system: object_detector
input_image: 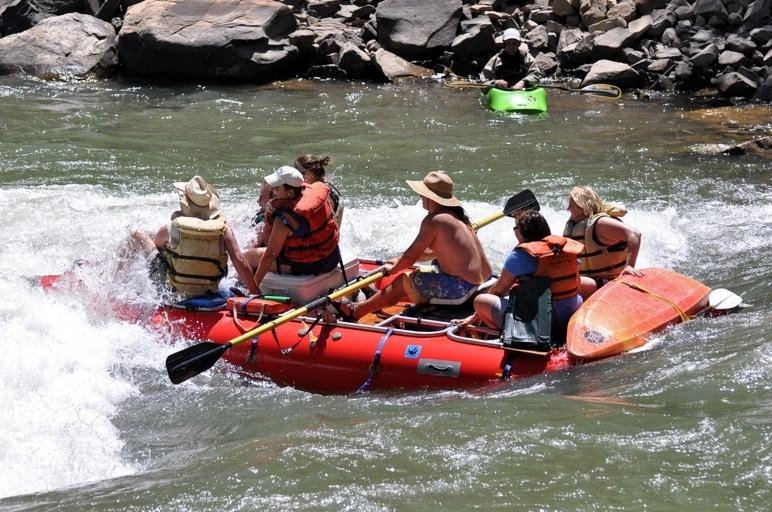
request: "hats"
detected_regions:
[263,165,305,188]
[405,168,463,210]
[172,174,220,219]
[502,26,523,43]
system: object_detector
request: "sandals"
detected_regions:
[356,276,377,299]
[328,287,360,324]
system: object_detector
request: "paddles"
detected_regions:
[442,79,621,99]
[689,287,743,318]
[164,188,542,383]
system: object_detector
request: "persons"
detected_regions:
[243,154,344,293]
[127,176,261,308]
[480,28,541,92]
[472,209,584,340]
[332,170,492,323]
[564,184,646,293]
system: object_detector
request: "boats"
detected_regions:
[34,262,714,398]
[479,85,549,116]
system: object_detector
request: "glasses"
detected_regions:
[513,226,519,230]
